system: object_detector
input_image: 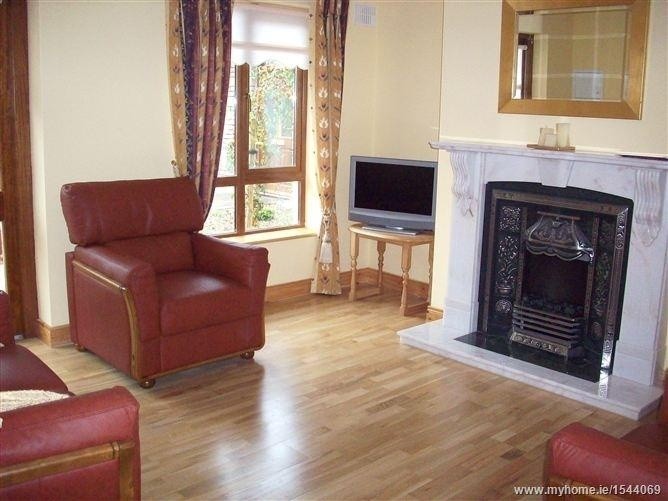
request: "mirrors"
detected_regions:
[497,0,651,120]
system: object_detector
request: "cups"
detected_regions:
[536,123,569,148]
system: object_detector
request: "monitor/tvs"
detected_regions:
[347,155,438,235]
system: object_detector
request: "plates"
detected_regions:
[527,143,576,152]
[617,151,668,162]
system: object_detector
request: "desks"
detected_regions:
[348,224,434,318]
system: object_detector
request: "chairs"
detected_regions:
[543,370,668,501]
[60,177,270,391]
[0,288,143,501]
